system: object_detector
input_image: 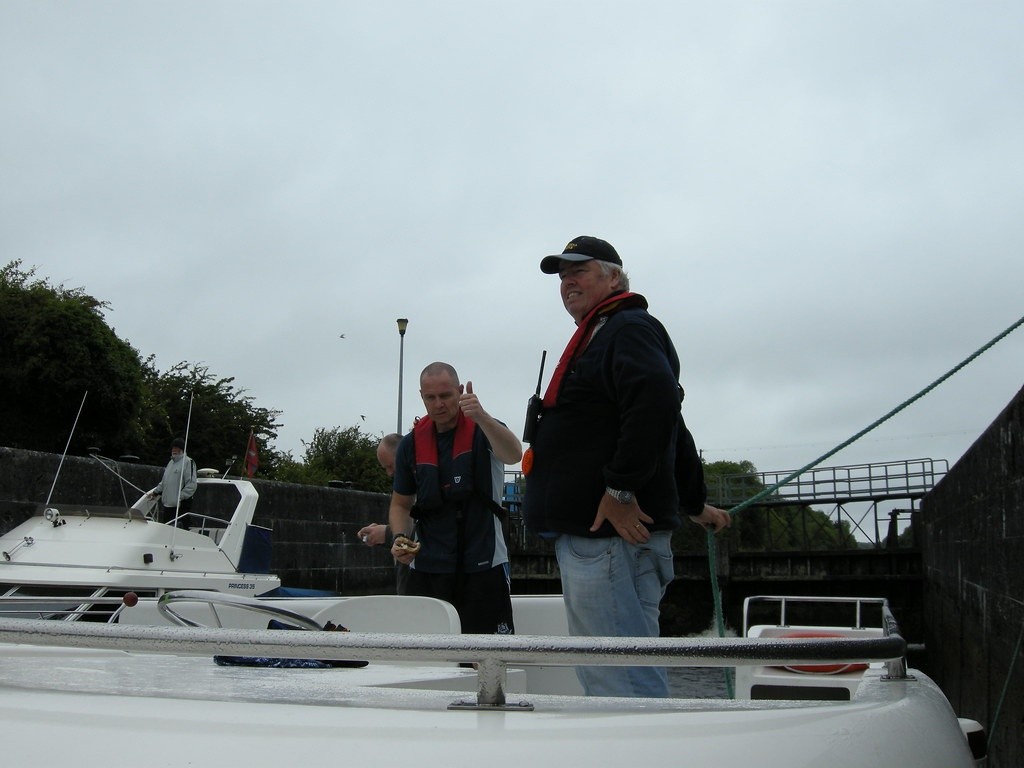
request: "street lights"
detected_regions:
[396,318,408,434]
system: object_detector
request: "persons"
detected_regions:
[149,438,197,530]
[522,236,731,697]
[357,433,418,595]
[388,362,522,636]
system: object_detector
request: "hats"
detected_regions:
[169,439,183,448]
[541,237,622,271]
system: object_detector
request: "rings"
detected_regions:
[635,522,641,527]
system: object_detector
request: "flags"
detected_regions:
[248,436,259,475]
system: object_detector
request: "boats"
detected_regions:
[1,585,990,768]
[0,467,463,666]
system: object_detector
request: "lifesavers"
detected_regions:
[765,633,869,674]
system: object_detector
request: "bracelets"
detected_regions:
[392,533,409,541]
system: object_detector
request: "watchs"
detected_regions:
[605,486,632,504]
